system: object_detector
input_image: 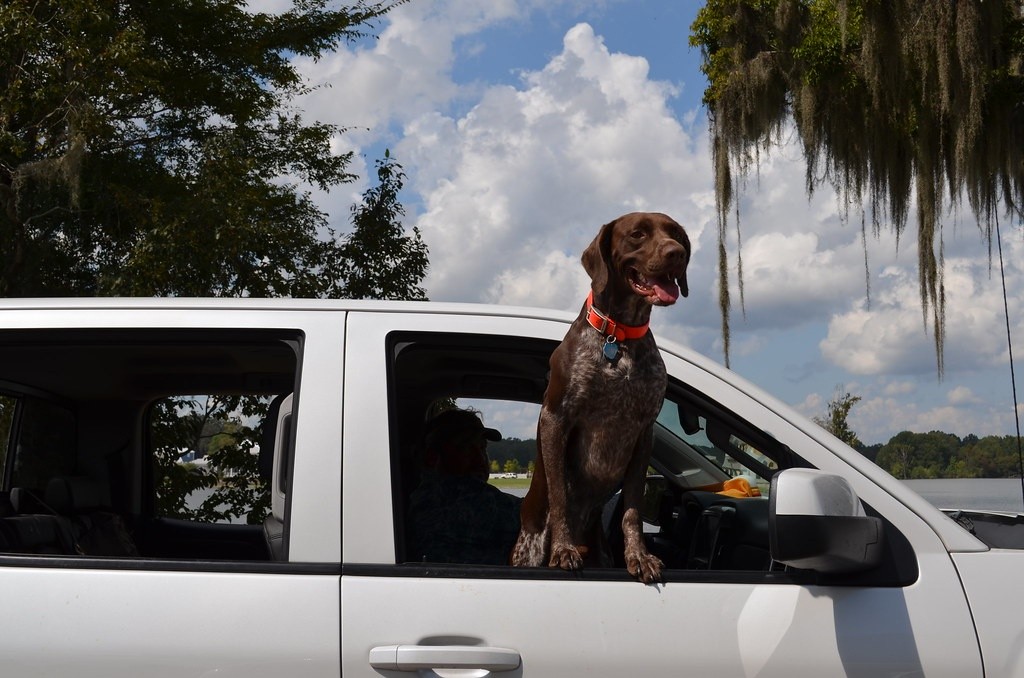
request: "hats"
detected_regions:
[424,409,501,455]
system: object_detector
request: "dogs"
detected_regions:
[507,207,693,588]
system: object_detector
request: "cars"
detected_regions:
[499,473,518,479]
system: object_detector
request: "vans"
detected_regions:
[0,296,1024,678]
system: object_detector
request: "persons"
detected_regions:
[404,410,525,566]
